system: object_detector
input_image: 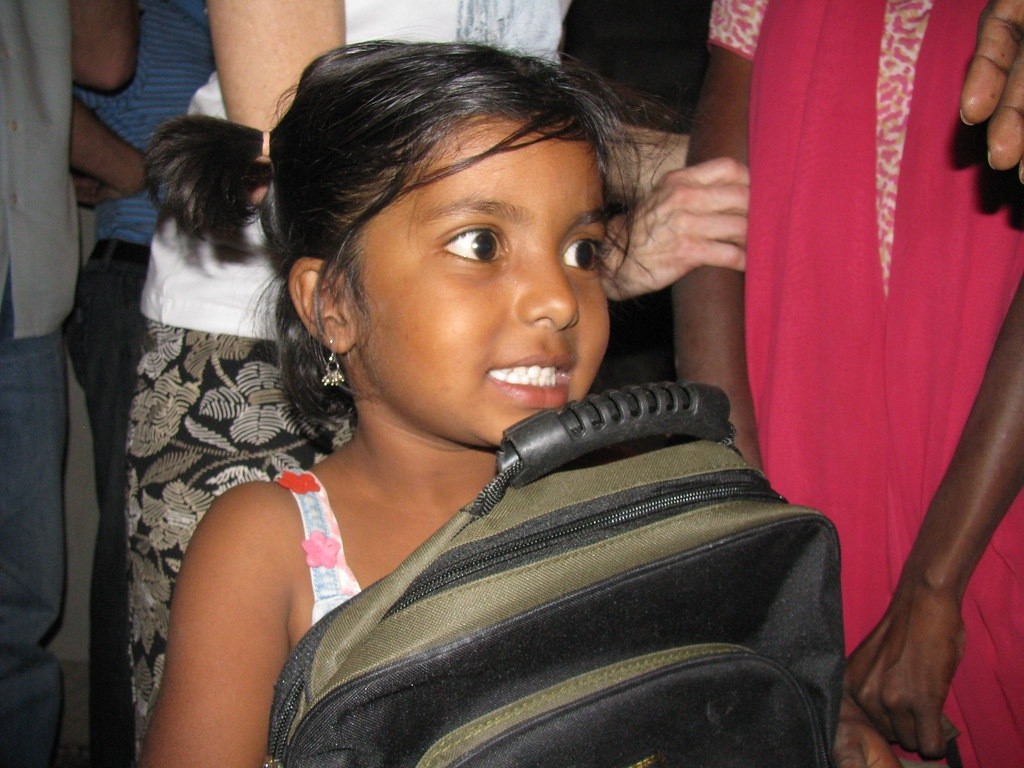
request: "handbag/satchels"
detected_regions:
[269,383,846,768]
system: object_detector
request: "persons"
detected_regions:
[0,0,1024,768]
[140,39,902,768]
